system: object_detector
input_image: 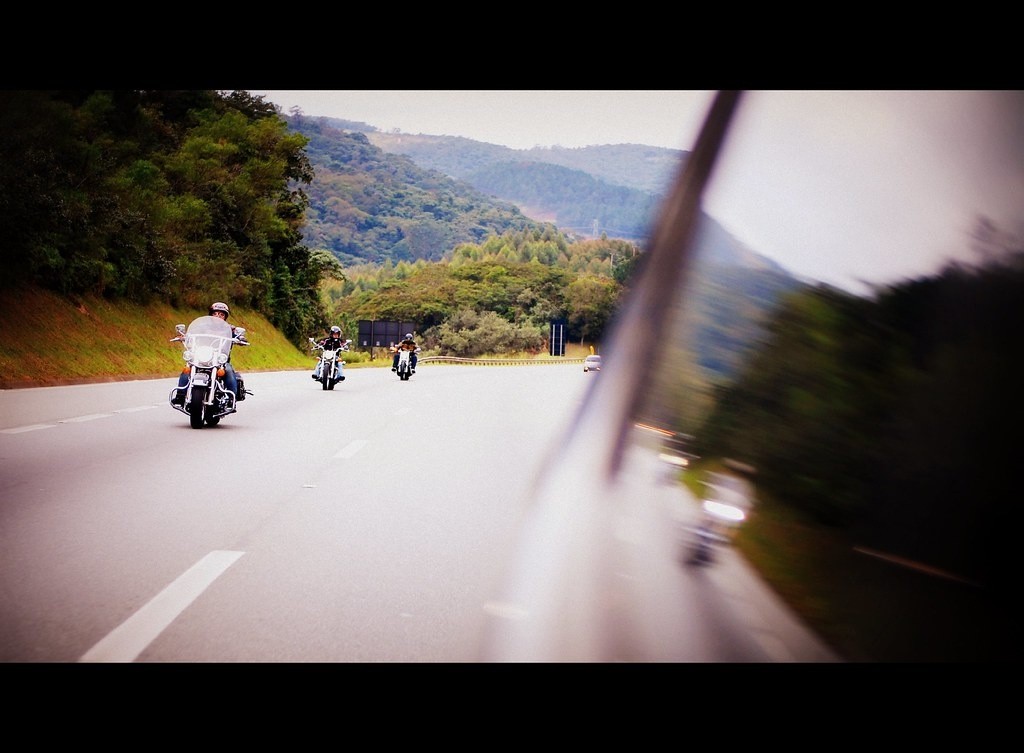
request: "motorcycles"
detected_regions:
[389,342,422,380]
[168,324,251,428]
[682,452,768,574]
[308,335,352,391]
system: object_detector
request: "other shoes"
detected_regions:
[171,394,185,408]
[412,370,415,373]
[336,376,345,381]
[392,369,396,371]
[312,374,319,379]
[227,397,236,409]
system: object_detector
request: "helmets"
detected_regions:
[330,326,341,337]
[405,333,413,339]
[209,302,229,317]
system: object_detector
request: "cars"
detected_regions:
[584,355,602,373]
[660,424,704,475]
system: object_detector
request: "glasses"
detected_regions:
[212,312,226,318]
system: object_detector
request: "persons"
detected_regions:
[171,302,248,407]
[391,333,421,373]
[312,326,349,381]
